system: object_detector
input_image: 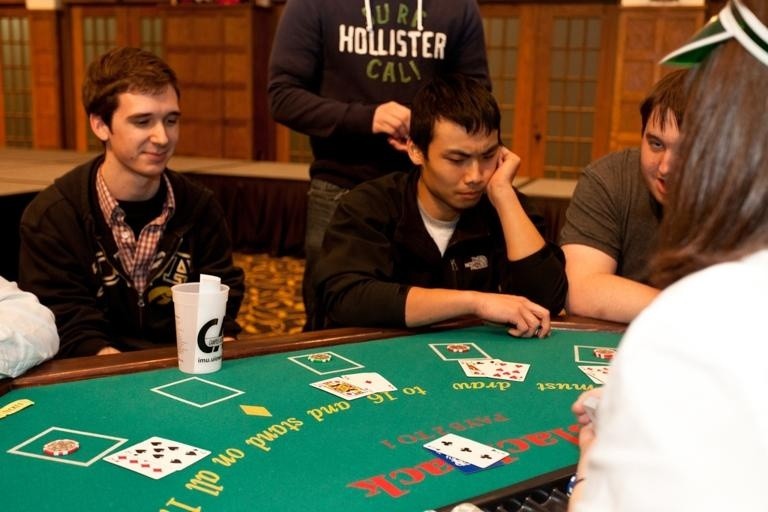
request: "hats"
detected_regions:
[657,0,768,68]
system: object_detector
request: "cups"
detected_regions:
[171,282,229,374]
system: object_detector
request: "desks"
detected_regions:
[0,314,640,512]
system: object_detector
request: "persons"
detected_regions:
[269,1,492,331]
[312,77,566,338]
[559,68,697,325]
[565,0,768,512]
[18,47,245,356]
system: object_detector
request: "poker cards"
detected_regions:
[577,365,610,384]
[459,358,531,382]
[102,436,211,480]
[423,432,511,472]
[308,372,398,402]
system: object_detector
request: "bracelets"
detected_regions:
[565,475,585,497]
